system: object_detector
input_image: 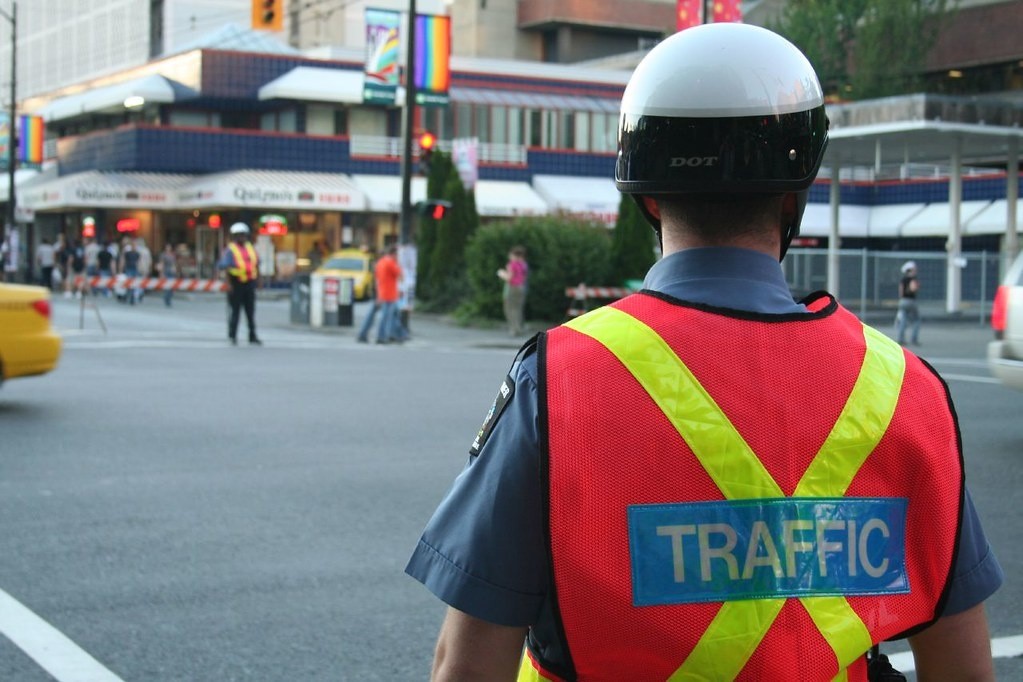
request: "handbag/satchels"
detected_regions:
[895,311,907,329]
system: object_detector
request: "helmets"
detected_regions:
[230,222,249,234]
[615,22,831,196]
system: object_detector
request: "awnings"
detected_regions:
[64,172,193,209]
[16,169,96,212]
[353,173,550,217]
[798,198,1023,236]
[532,174,621,229]
[0,157,59,202]
[161,169,366,212]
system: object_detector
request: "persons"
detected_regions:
[156,242,182,308]
[355,244,411,345]
[59,235,153,305]
[496,245,528,339]
[217,222,264,346]
[36,239,56,291]
[404,22,1004,682]
[306,238,325,271]
[895,260,921,345]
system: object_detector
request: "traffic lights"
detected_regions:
[419,200,455,221]
[418,132,434,176]
[252,0,284,30]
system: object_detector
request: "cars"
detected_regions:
[316,248,376,301]
[0,283,61,385]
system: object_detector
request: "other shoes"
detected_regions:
[899,341,909,346]
[230,337,239,345]
[251,337,262,345]
[911,341,921,346]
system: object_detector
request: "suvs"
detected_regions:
[987,250,1023,385]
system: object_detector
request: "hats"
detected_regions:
[901,261,915,274]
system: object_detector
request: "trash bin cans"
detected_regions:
[290,273,310,325]
